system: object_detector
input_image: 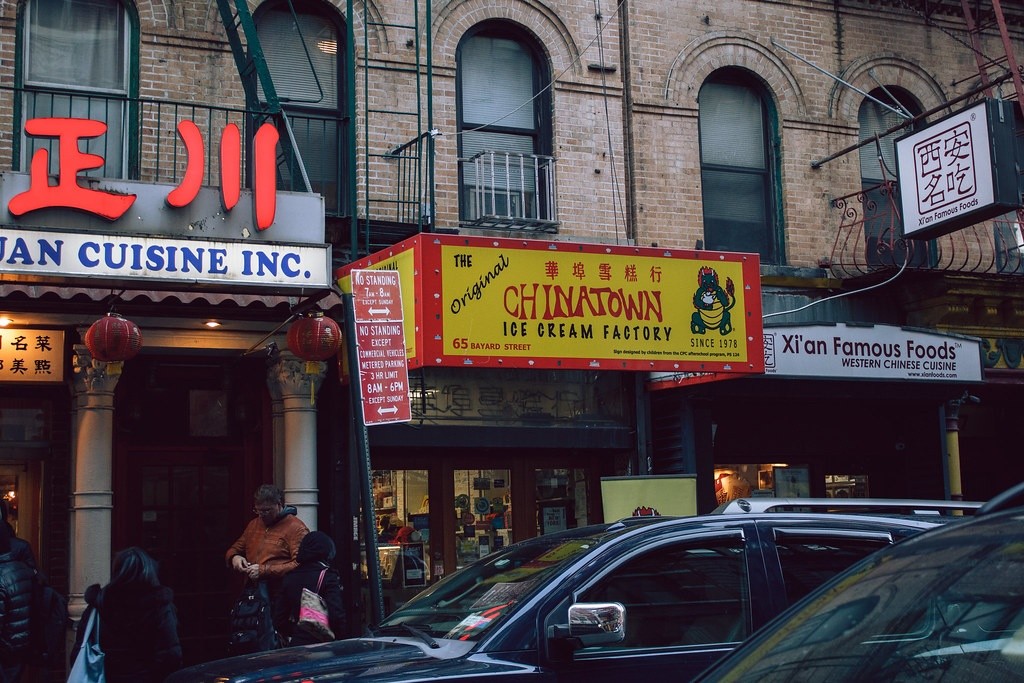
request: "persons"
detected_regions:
[225,483,313,653]
[0,528,39,683]
[273,528,348,643]
[0,518,34,568]
[67,547,187,683]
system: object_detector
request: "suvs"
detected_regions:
[173,488,990,683]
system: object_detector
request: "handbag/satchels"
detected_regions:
[65,608,106,683]
[299,567,335,643]
[230,578,277,655]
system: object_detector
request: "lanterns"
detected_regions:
[85,310,143,376]
[286,307,342,406]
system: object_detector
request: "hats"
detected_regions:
[296,531,336,565]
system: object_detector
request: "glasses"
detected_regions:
[253,504,278,514]
